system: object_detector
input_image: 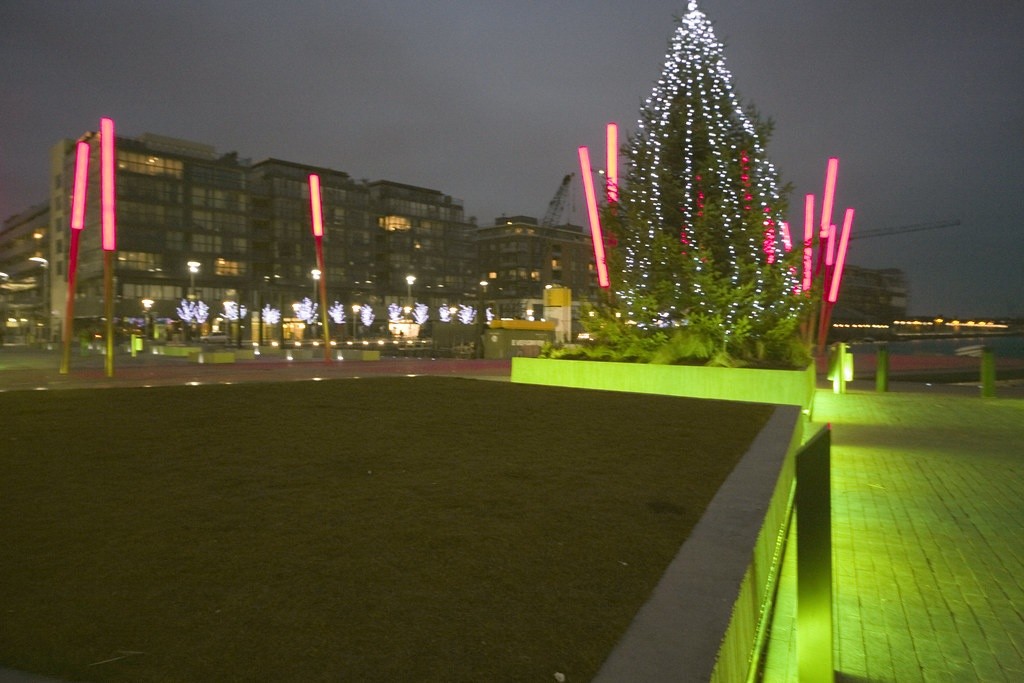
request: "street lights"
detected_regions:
[310,266,321,299]
[404,273,418,305]
[185,258,201,297]
